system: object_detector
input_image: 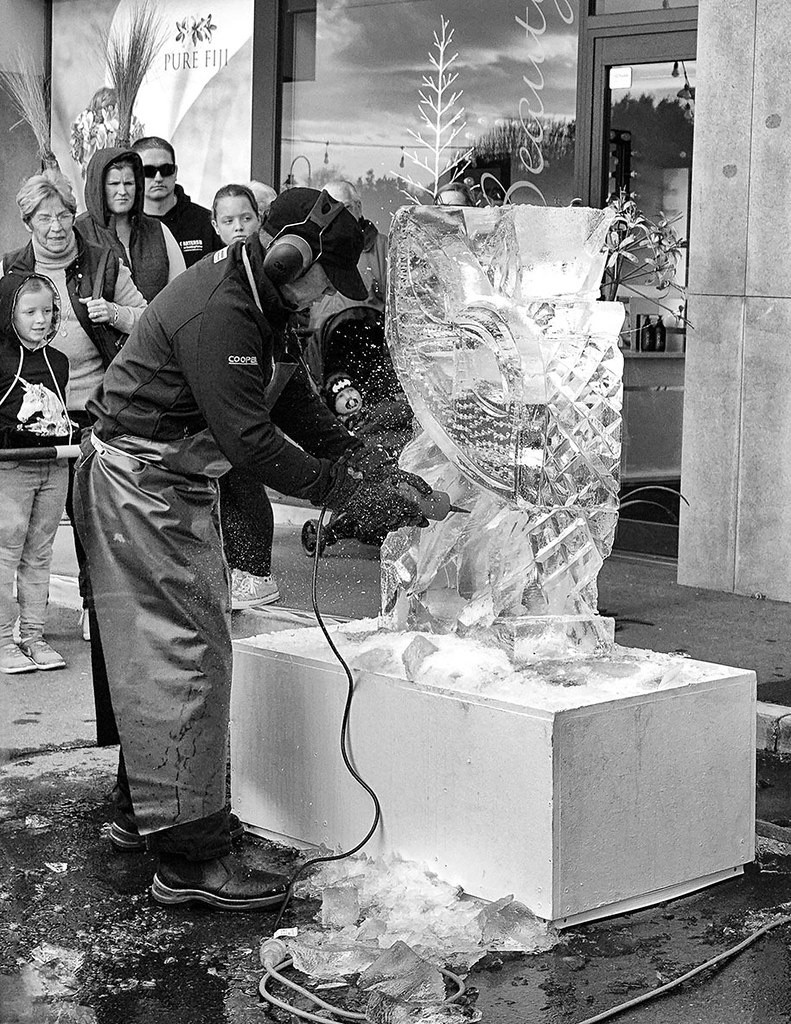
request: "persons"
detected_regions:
[0,136,474,641]
[73,186,431,908]
[0,270,70,674]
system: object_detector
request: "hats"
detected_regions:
[263,188,372,301]
[324,371,362,416]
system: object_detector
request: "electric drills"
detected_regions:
[302,479,470,558]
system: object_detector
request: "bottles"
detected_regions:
[641,315,667,353]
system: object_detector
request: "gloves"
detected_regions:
[316,447,433,545]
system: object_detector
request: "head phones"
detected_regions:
[264,189,343,287]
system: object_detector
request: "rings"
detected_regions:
[95,312,99,318]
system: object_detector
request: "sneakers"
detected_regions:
[228,568,281,610]
[0,636,66,675]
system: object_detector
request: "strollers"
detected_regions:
[296,290,424,558]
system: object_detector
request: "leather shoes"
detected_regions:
[109,811,242,850]
[153,856,292,910]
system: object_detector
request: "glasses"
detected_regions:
[29,213,74,225]
[143,163,178,178]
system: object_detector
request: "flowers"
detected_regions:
[550,184,696,331]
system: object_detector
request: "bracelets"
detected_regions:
[111,302,119,327]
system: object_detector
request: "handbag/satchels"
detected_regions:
[92,248,131,371]
